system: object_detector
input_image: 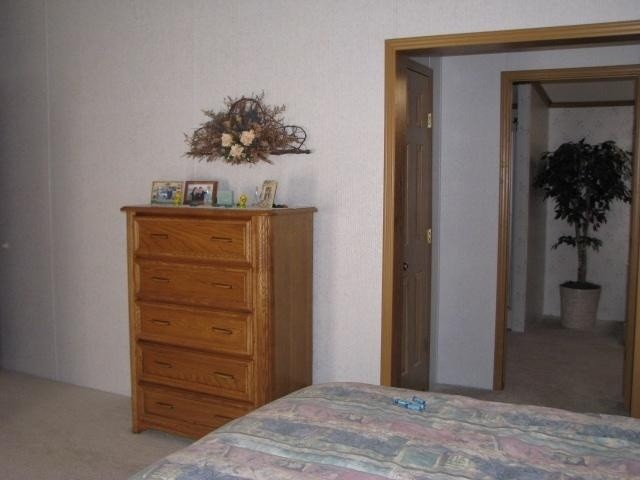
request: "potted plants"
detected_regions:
[532,137,633,331]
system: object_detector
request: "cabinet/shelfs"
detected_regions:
[120,203,318,440]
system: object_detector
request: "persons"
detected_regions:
[154,183,175,200]
[190,186,207,203]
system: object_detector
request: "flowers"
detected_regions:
[180,91,307,167]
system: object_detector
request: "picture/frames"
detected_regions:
[183,181,217,207]
[260,180,277,208]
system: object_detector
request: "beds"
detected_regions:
[126,381,640,480]
